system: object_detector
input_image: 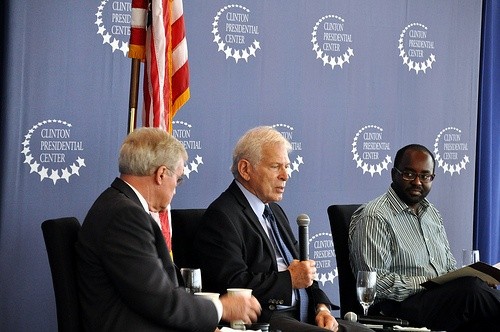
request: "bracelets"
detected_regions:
[317,307,331,313]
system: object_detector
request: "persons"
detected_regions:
[194,126,340,332]
[70,127,262,332]
[348,144,500,332]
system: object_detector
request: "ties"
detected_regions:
[263,205,309,325]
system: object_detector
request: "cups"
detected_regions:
[194,292,221,300]
[181,267,202,294]
[244,323,270,332]
[462,249,479,268]
[227,288,253,329]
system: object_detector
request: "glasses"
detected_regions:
[394,166,434,184]
[155,164,184,187]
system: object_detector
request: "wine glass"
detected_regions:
[357,270,377,316]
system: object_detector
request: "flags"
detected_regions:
[142,0,190,136]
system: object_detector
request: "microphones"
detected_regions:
[296,214,310,261]
[345,311,410,326]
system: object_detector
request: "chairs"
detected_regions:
[42,216,84,332]
[170,209,207,286]
[327,204,367,321]
